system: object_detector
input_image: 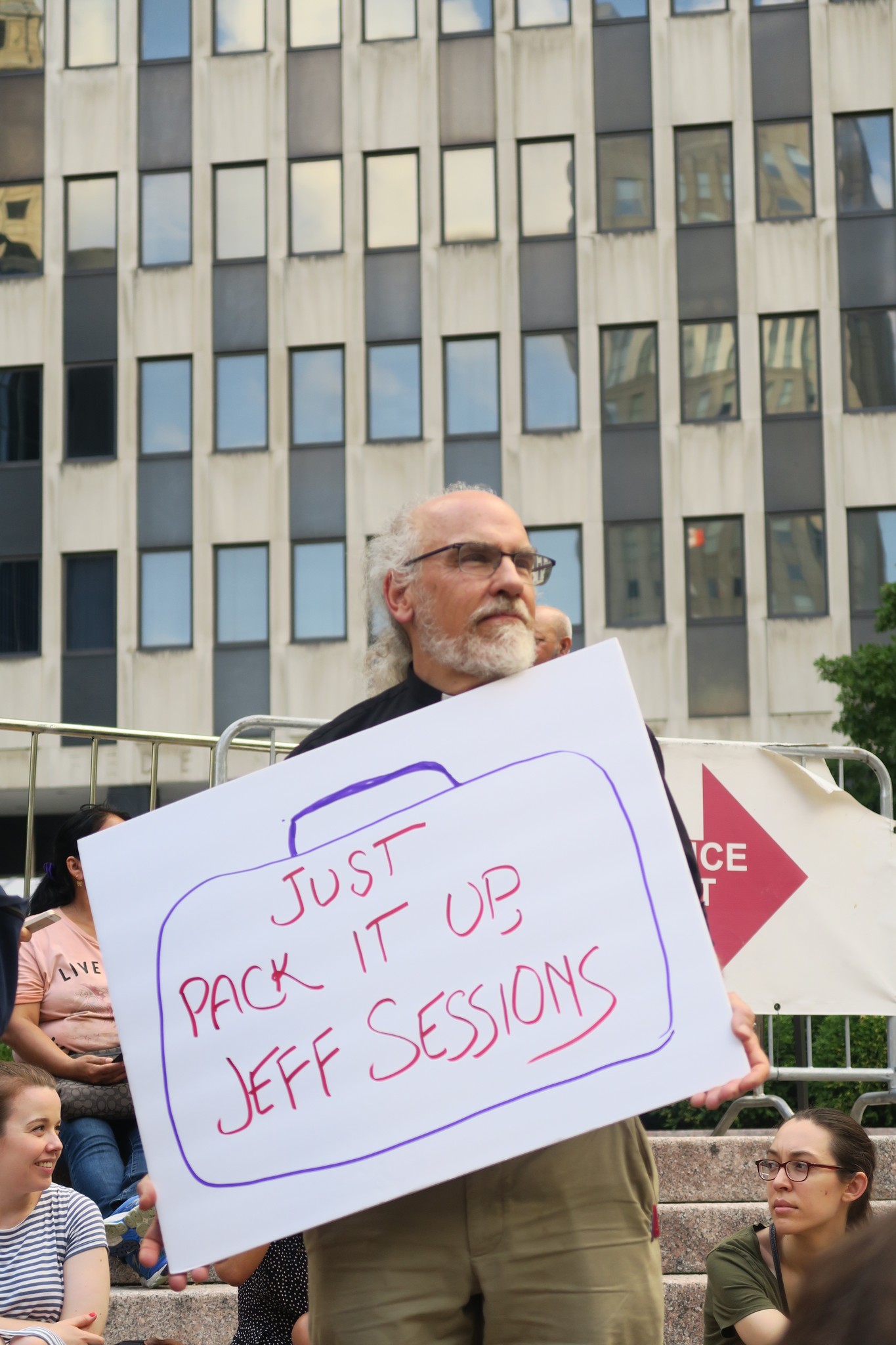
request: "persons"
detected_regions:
[533,606,572,666]
[137,480,772,1345]
[0,1061,111,1345]
[703,1108,877,1345]
[229,1232,311,1344]
[0,804,170,1289]
[769,1198,896,1345]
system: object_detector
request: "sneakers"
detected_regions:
[125,1248,170,1289]
[104,1194,159,1259]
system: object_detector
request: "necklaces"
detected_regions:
[769,1223,790,1319]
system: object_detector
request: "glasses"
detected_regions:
[755,1159,853,1183]
[404,540,557,587]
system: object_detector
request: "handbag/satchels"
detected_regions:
[53,1045,135,1119]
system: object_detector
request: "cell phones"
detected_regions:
[112,1052,123,1063]
